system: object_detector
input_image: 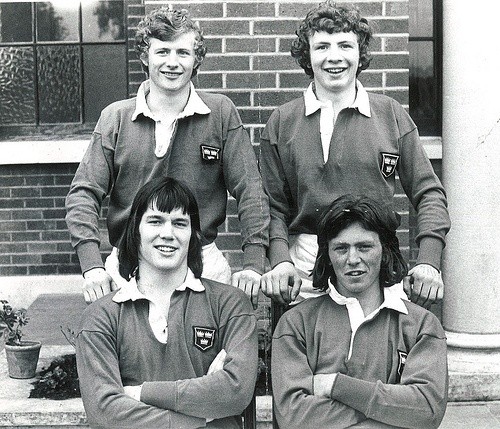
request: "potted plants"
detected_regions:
[0,300,42,378]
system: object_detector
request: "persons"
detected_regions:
[270,193,450,429]
[257,1,452,310]
[74,176,260,429]
[65,6,271,311]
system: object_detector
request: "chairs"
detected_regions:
[233,290,257,429]
[271,278,431,429]
